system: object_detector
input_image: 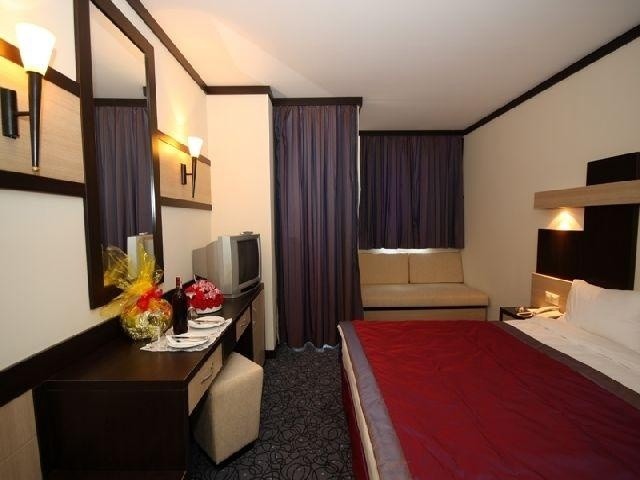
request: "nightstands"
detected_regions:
[498,304,563,324]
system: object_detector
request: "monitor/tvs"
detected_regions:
[191,233,262,299]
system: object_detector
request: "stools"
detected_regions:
[192,349,264,468]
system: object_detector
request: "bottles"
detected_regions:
[171,276,188,331]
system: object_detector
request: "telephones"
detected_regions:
[532,306,563,319]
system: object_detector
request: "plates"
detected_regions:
[169,335,207,349]
[192,317,225,328]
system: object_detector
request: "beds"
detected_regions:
[335,282,639,480]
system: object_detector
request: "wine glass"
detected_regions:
[185,283,196,320]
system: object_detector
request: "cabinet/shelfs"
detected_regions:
[32,282,267,479]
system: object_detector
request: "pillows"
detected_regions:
[563,277,639,354]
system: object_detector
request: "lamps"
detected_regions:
[181,136,205,202]
[1,19,58,173]
[545,205,584,233]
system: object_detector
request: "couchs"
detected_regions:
[356,249,490,322]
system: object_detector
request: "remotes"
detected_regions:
[240,231,253,236]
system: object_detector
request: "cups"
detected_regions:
[150,330,167,349]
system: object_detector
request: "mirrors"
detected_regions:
[67,0,170,313]
